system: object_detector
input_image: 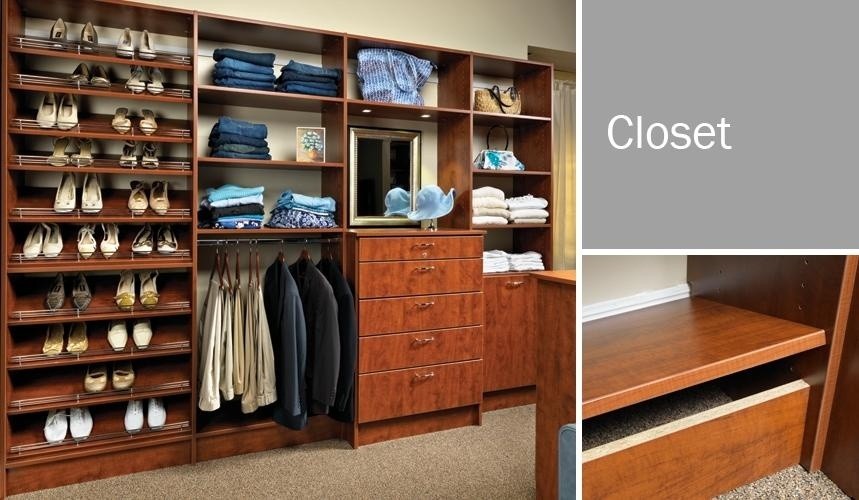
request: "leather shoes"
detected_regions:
[125,66,146,94]
[81,21,99,53]
[146,66,165,95]
[50,16,68,48]
[91,65,111,87]
[73,62,91,86]
[118,29,135,58]
[139,29,156,61]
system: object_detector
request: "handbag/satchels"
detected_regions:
[474,125,525,172]
[356,47,439,108]
[475,84,522,116]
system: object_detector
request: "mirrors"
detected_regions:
[348,124,423,229]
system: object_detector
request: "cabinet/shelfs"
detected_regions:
[526,271,577,497]
[0,0,555,500]
[582,256,859,500]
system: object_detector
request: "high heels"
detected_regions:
[35,92,160,169]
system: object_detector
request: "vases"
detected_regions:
[307,149,317,160]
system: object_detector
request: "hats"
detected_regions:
[384,186,412,217]
[406,185,457,222]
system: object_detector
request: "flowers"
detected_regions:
[301,131,323,154]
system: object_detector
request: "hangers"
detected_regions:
[208,237,334,302]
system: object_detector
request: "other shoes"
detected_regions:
[41,270,166,444]
[22,172,178,259]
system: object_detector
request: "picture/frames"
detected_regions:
[296,125,326,165]
